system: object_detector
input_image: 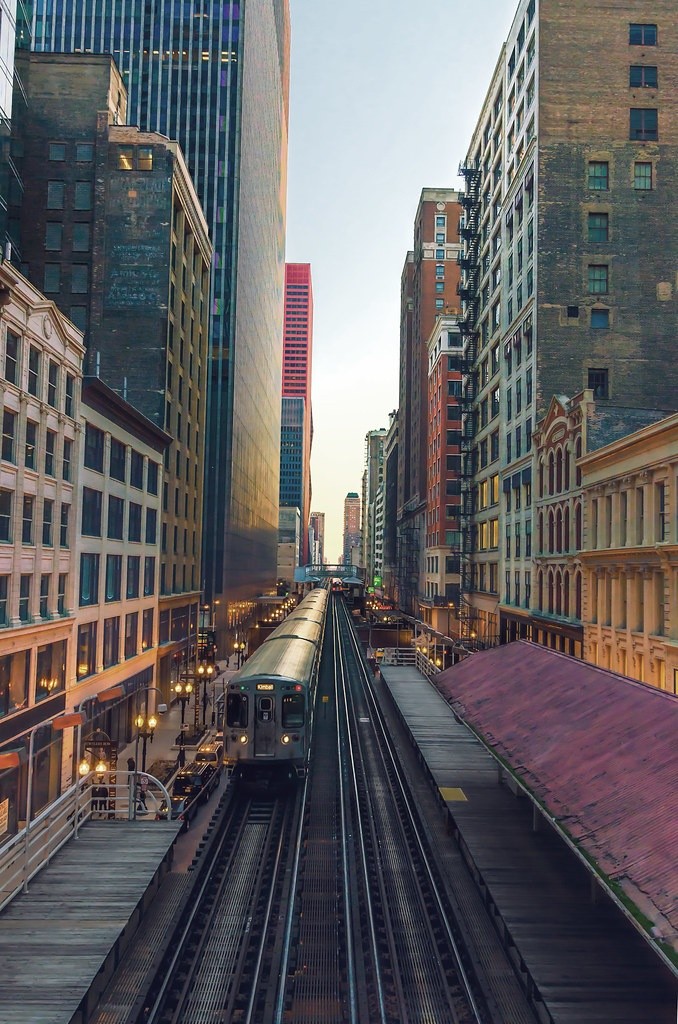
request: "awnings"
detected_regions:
[424,639,678,979]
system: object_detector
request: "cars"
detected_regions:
[193,743,224,772]
[172,763,220,803]
[211,732,223,744]
[155,796,199,831]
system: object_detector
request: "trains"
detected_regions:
[223,588,329,784]
[331,578,343,594]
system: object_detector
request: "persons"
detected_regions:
[215,664,220,678]
[91,782,108,816]
[127,757,135,784]
[201,693,212,711]
[226,655,229,667]
[241,653,248,667]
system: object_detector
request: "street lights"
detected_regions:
[290,598,295,610]
[74,685,126,840]
[174,683,192,767]
[133,687,167,820]
[234,642,245,670]
[23,710,87,895]
[135,715,157,772]
[448,603,453,637]
[285,602,290,616]
[200,602,209,663]
[288,601,293,610]
[198,665,213,725]
[281,605,287,619]
